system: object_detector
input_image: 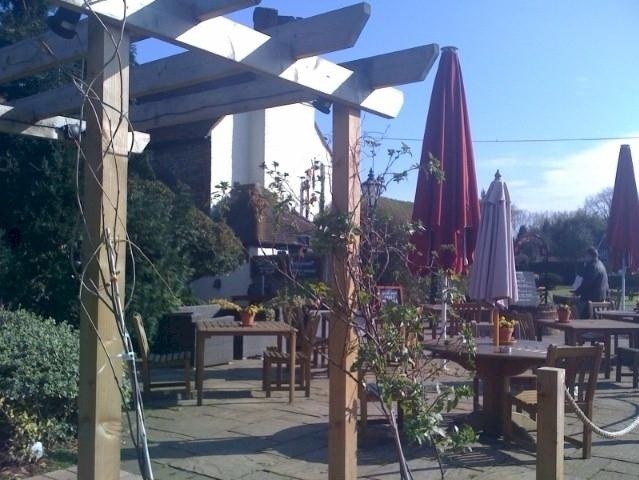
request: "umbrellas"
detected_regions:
[603,142,639,311]
[467,168,519,350]
[403,45,484,364]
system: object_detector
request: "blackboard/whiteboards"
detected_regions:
[375,286,405,307]
[512,270,537,307]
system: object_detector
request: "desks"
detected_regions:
[319,311,367,362]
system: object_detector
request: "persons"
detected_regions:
[570,245,609,318]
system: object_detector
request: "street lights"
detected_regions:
[361,168,381,343]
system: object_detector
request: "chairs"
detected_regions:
[131,307,328,405]
